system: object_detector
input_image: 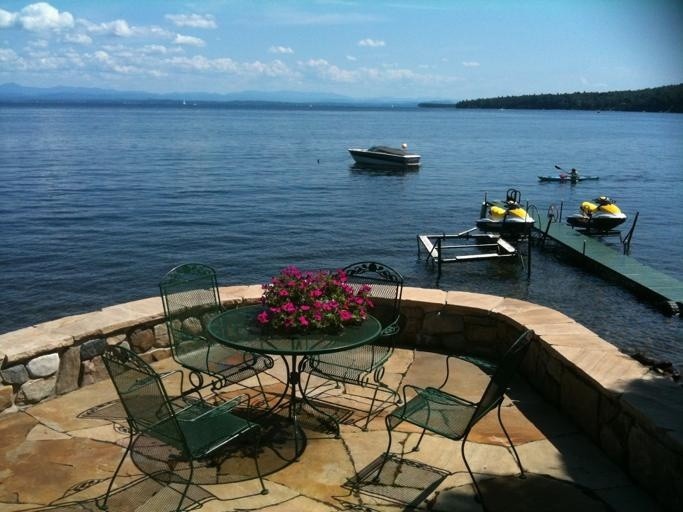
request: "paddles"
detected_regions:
[554,165,568,174]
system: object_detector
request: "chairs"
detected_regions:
[100,345,270,512]
[158,261,405,462]
[372,330,535,510]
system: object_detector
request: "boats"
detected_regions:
[348,144,422,172]
[537,168,600,183]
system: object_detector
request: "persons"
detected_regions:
[564,168,581,182]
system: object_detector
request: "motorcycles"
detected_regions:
[476,198,535,231]
[566,196,627,231]
